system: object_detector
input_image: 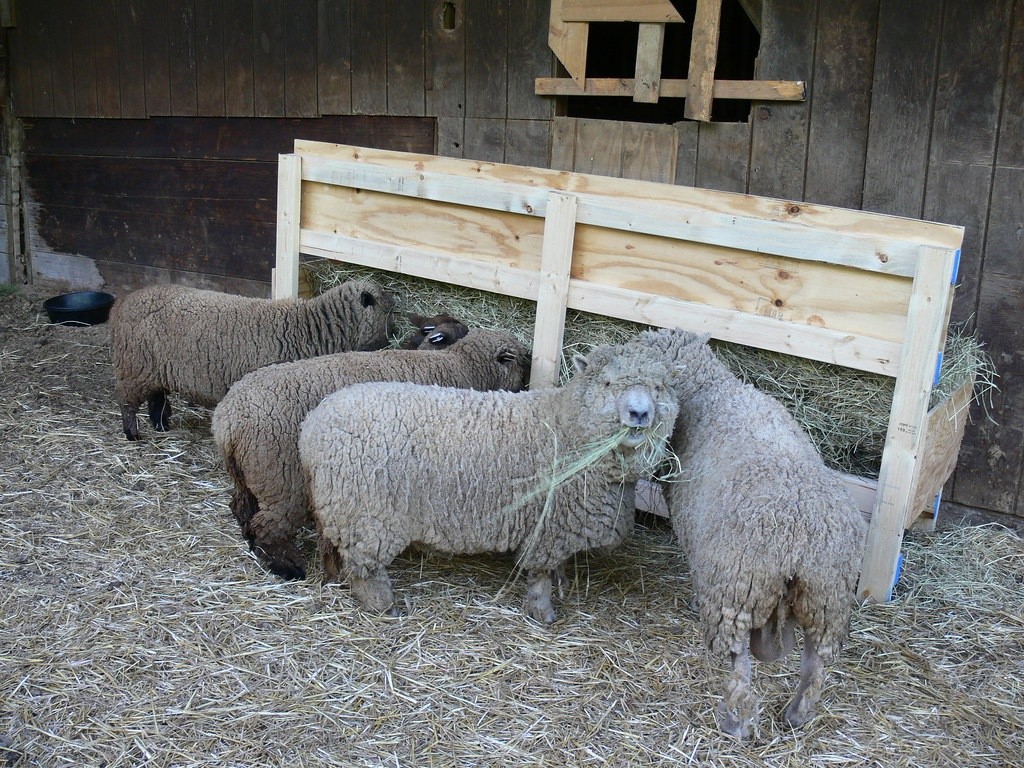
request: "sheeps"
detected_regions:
[629,324,869,741]
[107,278,471,444]
[297,342,686,624]
[212,325,528,582]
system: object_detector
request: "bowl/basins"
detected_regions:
[44,291,116,328]
